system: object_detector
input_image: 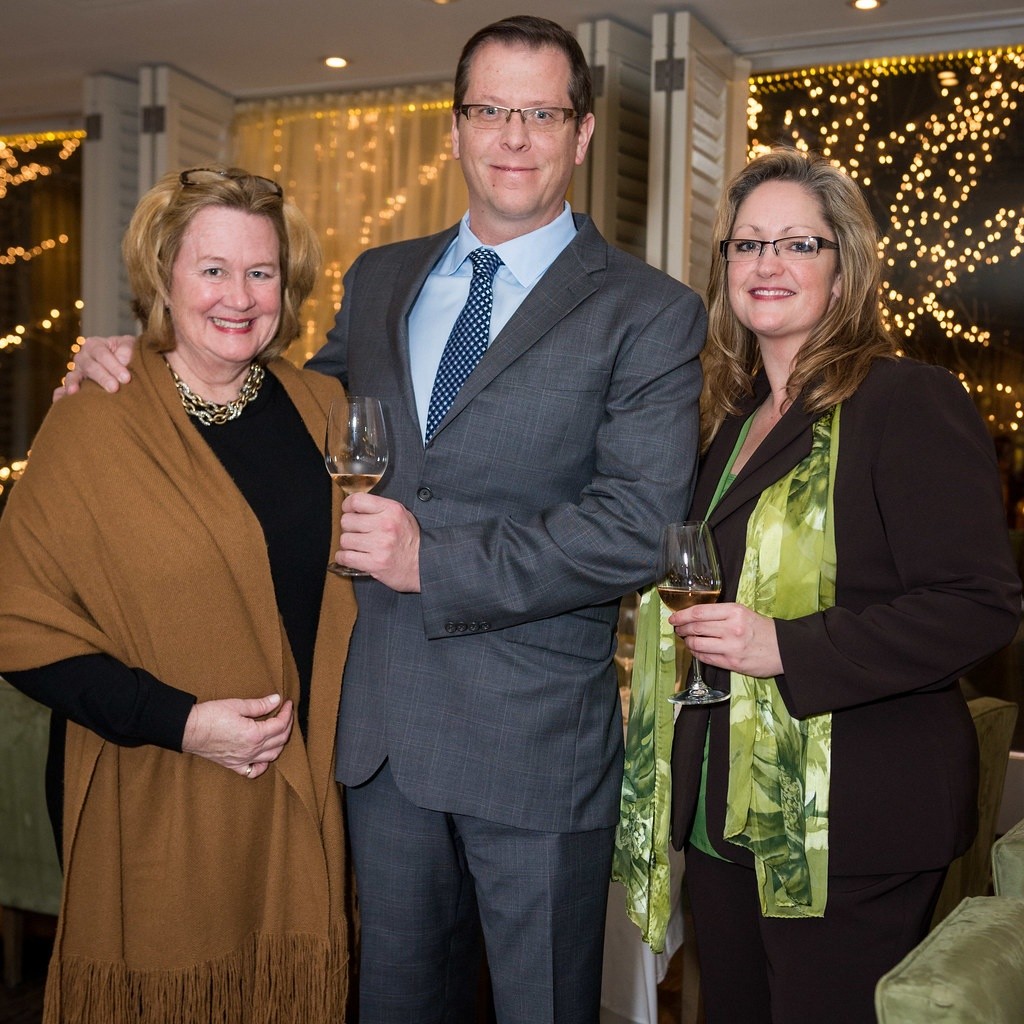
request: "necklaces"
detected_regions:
[160,361,266,426]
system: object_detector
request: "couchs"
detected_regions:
[0,594,1024,1024]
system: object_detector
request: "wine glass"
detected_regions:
[655,520,730,704]
[325,398,389,576]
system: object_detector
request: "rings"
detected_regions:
[242,765,253,778]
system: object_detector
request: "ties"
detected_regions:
[425,246,505,450]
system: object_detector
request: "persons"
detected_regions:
[611,147,1024,1024]
[0,163,357,1024]
[53,15,707,1024]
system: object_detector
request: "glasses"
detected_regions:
[459,104,581,132]
[168,169,283,215]
[719,235,839,262]
